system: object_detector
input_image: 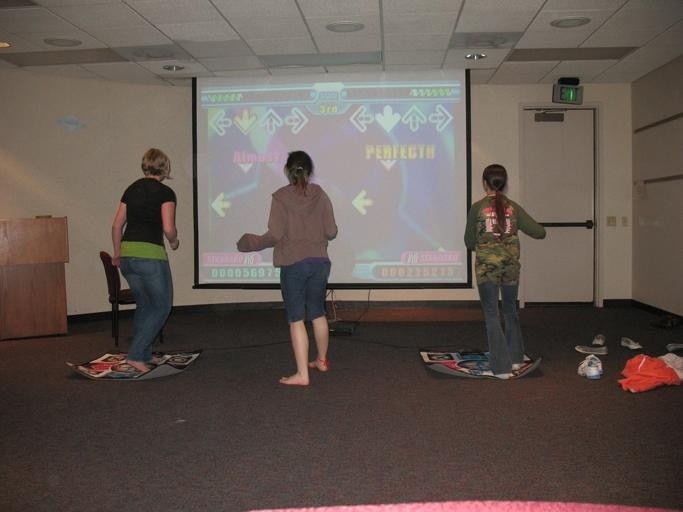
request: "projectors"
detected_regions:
[328,321,360,335]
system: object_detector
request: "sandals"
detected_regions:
[620,337,643,349]
[666,343,683,353]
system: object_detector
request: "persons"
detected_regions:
[236,151,338,387]
[464,164,546,379]
[111,148,179,374]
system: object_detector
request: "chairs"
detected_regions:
[98,248,166,347]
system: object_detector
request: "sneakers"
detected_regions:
[575,334,608,380]
[649,316,676,329]
